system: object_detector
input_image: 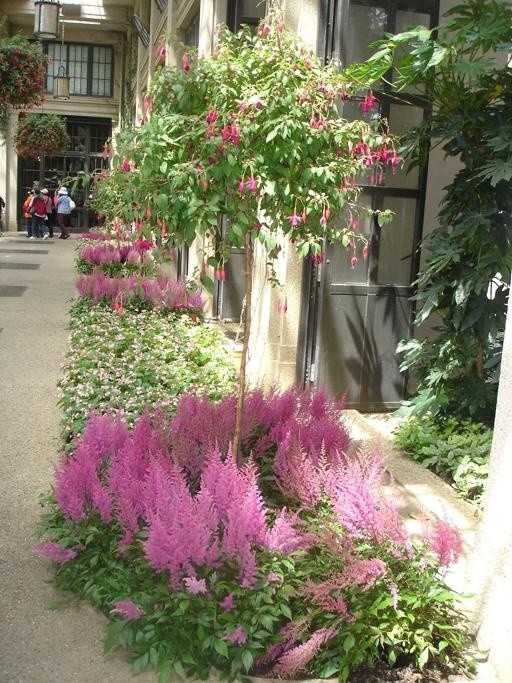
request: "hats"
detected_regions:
[58,186,69,194]
[40,187,49,195]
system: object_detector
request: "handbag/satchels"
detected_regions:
[68,196,76,210]
[25,212,33,218]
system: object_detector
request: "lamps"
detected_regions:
[52,40,71,101]
[33,0,63,40]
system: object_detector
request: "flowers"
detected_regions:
[1,28,48,144]
[15,113,69,160]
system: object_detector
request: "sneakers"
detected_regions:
[26,233,54,240]
[59,234,70,240]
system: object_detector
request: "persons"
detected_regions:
[56,187,72,239]
[23,188,54,240]
